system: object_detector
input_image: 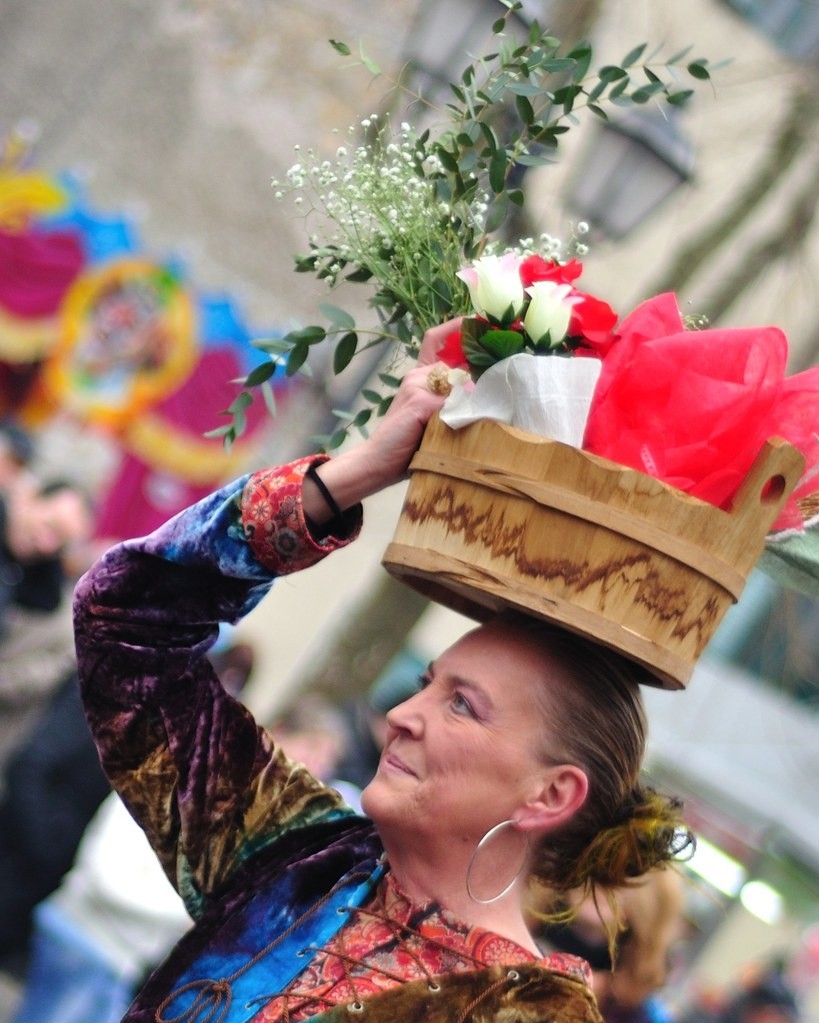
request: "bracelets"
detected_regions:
[308,468,343,519]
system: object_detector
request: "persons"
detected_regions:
[1,427,90,650]
[9,694,356,1023]
[338,675,420,792]
[521,862,683,1023]
[0,618,255,978]
[72,314,700,1023]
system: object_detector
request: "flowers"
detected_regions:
[204,0,739,450]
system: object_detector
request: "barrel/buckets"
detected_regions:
[381,409,807,693]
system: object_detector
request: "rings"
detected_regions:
[425,367,452,395]
